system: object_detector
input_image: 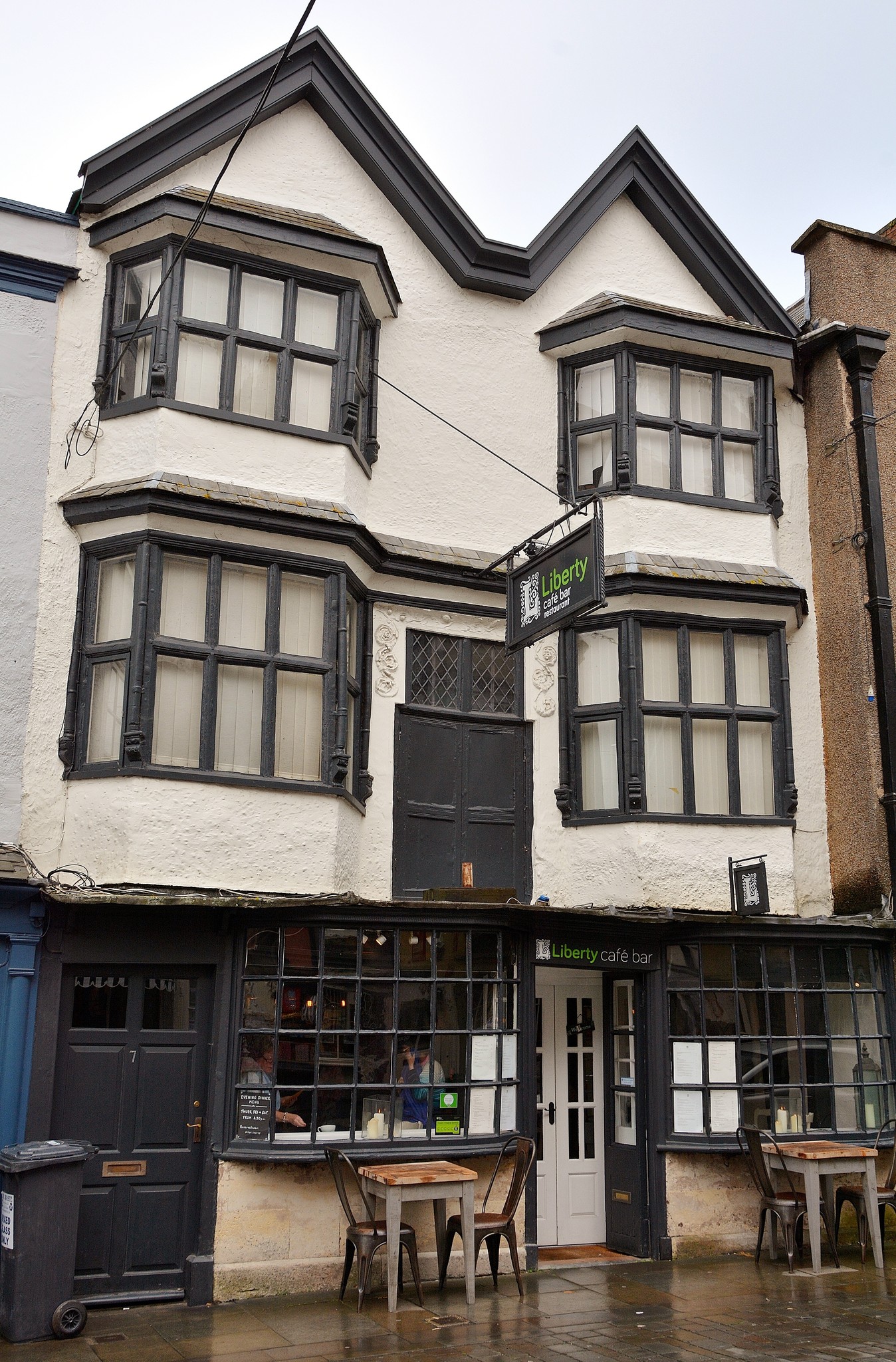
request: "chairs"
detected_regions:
[320,1141,427,1311]
[436,1129,540,1298]
[734,1124,843,1273]
[826,1118,896,1265]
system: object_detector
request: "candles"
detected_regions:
[798,1114,803,1132]
[775,1121,782,1133]
[783,1107,789,1123]
[865,1103,876,1129]
[777,1106,787,1133]
[790,1113,798,1133]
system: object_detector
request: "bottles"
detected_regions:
[535,895,550,906]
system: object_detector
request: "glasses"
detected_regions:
[400,1044,414,1053]
[258,1053,273,1063]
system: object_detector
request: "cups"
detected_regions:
[318,1125,336,1132]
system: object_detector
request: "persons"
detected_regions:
[243,1037,306,1132]
[397,1034,445,1131]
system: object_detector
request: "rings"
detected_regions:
[297,1126,299,1127]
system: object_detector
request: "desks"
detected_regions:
[761,1139,885,1274]
[354,1157,478,1313]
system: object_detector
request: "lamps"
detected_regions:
[409,931,419,945]
[361,930,368,945]
[376,930,387,946]
[426,931,432,946]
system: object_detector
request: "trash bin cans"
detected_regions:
[0,1139,100,1344]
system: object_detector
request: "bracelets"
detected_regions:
[284,1112,286,1123]
